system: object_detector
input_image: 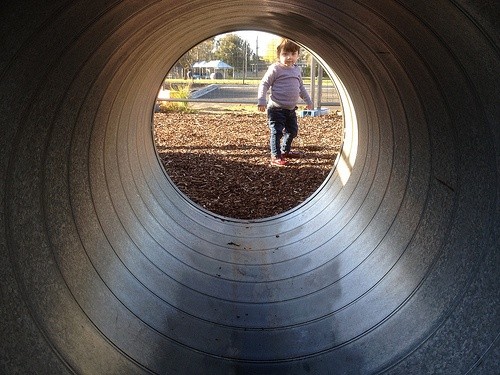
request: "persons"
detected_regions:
[257,37,313,166]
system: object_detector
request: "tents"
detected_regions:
[192,59,231,80]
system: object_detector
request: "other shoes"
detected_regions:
[281,153,293,159]
[271,157,289,166]
